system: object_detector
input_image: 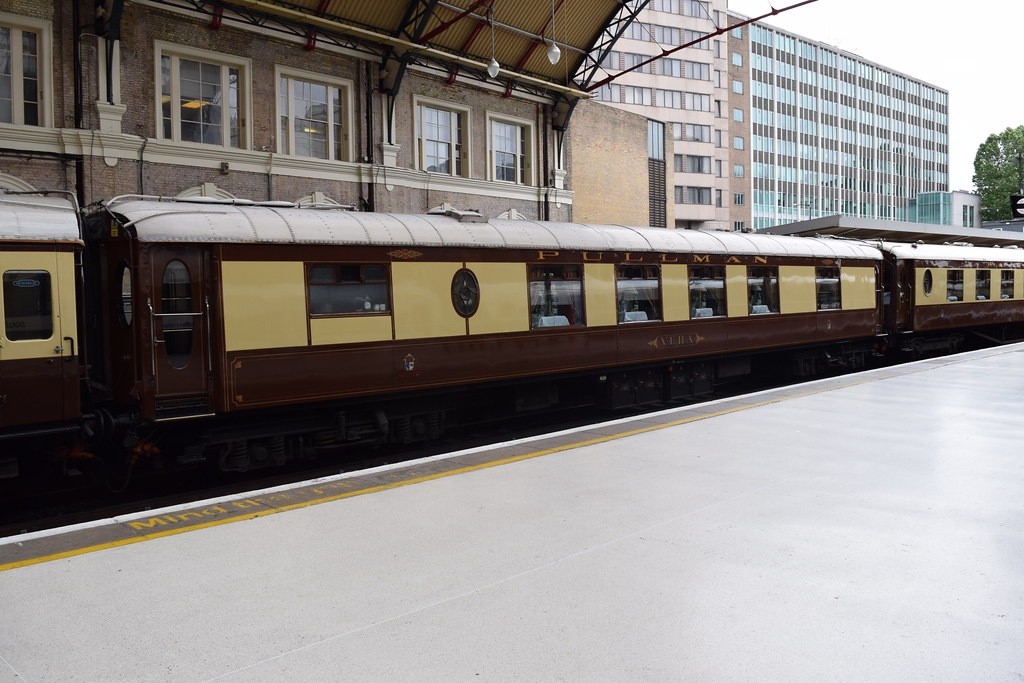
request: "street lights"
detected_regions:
[793,203,811,220]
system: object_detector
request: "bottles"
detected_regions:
[702,294,706,308]
[758,293,762,305]
[553,300,558,315]
[364,296,372,312]
[634,296,639,311]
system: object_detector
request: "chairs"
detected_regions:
[694,308,713,317]
[751,305,770,314]
[538,315,570,327]
[623,311,648,322]
[948,294,1009,301]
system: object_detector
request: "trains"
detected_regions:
[0,191,1024,458]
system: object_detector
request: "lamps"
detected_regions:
[547,0,561,65]
[487,5,499,79]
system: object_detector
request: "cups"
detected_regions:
[373,304,379,311]
[380,304,385,310]
